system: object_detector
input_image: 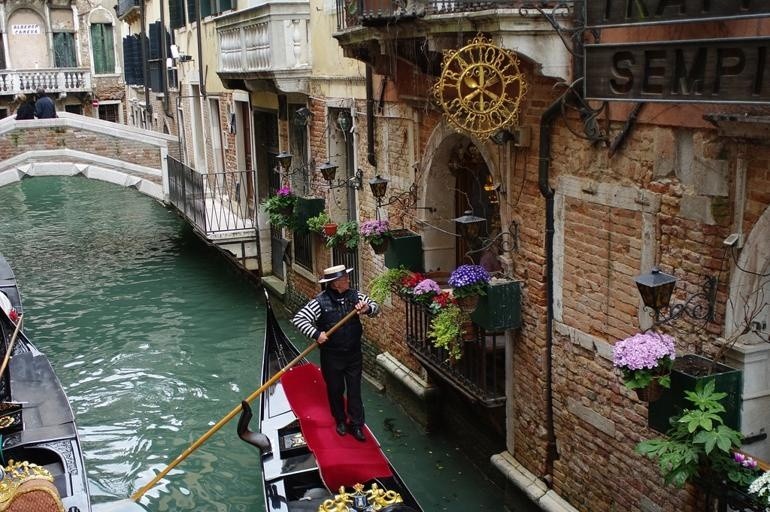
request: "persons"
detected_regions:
[292,264,379,442]
[14,94,35,120]
[34,89,57,118]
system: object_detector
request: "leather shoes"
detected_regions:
[336,420,346,435]
[348,424,365,440]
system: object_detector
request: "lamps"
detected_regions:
[634,268,715,326]
[451,210,518,258]
[272,151,417,210]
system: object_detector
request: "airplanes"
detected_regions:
[0,248,94,512]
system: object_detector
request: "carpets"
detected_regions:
[279,361,394,495]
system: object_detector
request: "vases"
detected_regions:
[324,223,338,236]
[462,319,477,342]
[370,239,388,255]
[278,205,293,215]
[648,353,742,446]
[633,376,663,402]
[457,294,479,314]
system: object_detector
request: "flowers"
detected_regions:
[360,219,392,246]
[366,264,464,365]
[447,264,490,300]
[720,452,770,508]
[613,332,677,390]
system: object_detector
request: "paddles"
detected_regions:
[133,301,366,503]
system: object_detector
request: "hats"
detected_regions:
[317,265,353,284]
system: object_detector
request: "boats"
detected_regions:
[238,288,424,512]
